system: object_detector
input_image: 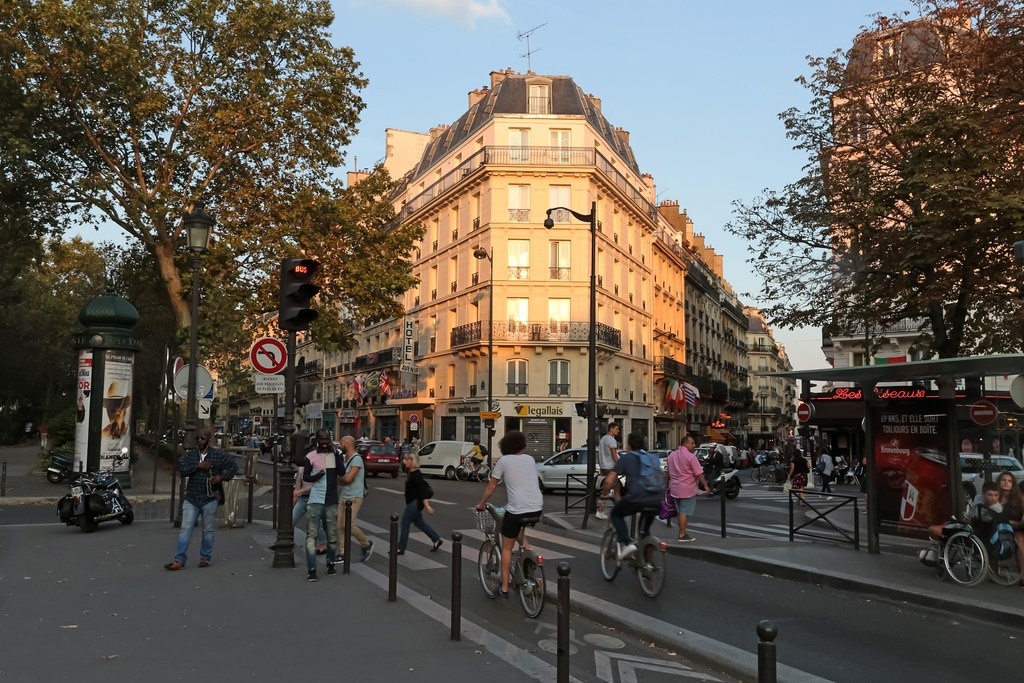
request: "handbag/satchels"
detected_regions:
[815,456,826,473]
[783,474,792,494]
[659,487,677,520]
[418,480,433,511]
[364,484,369,497]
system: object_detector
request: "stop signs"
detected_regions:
[796,403,811,422]
[969,400,999,425]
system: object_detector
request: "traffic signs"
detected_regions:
[480,411,502,419]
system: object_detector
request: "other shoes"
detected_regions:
[326,561,336,577]
[594,510,608,520]
[818,495,822,499]
[307,568,318,582]
[199,559,209,567]
[359,540,375,562]
[491,588,509,601]
[860,509,867,514]
[826,496,834,501]
[796,501,806,507]
[677,533,695,543]
[387,549,404,555]
[619,545,638,560]
[164,560,185,570]
[316,548,327,556]
[334,555,345,564]
[430,540,443,552]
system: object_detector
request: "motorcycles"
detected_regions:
[56,447,135,534]
[697,458,742,499]
[42,448,75,484]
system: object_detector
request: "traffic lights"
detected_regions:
[295,381,318,405]
[278,257,323,331]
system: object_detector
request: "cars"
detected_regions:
[331,440,400,480]
[212,432,285,453]
[759,449,781,465]
[536,445,673,495]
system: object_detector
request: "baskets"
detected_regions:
[471,510,505,534]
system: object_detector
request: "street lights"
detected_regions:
[172,198,218,528]
[544,201,597,512]
[473,245,493,470]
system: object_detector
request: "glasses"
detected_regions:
[195,436,205,441]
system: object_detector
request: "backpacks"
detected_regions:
[479,445,488,456]
[976,503,1016,560]
[630,449,665,493]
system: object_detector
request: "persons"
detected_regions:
[160,423,486,581]
[474,431,544,602]
[102,383,131,439]
[595,422,1024,591]
[24,420,49,450]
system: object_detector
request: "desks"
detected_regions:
[831,464,846,484]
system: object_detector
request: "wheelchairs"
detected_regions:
[920,514,1024,588]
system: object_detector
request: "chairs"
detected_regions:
[844,462,860,486]
[813,467,823,484]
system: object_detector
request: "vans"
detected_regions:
[415,441,488,480]
[692,443,751,469]
[958,451,1024,498]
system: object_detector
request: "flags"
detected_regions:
[667,379,699,411]
[353,370,393,403]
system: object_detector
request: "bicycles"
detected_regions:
[599,494,667,598]
[469,504,547,619]
[750,468,790,486]
[455,454,505,485]
